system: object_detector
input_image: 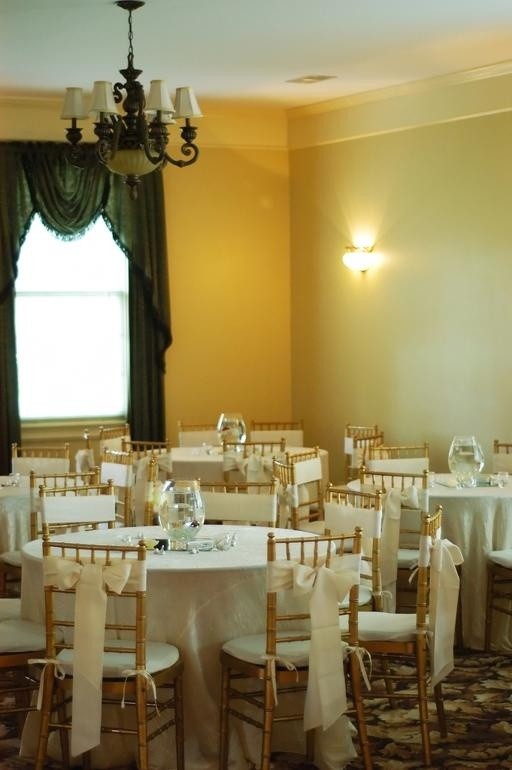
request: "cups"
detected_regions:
[497,471,509,488]
[426,472,435,487]
[214,530,237,551]
[9,473,21,488]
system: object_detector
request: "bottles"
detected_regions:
[448,435,485,490]
[218,411,246,453]
[157,479,206,551]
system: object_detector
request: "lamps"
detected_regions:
[60,0,205,200]
